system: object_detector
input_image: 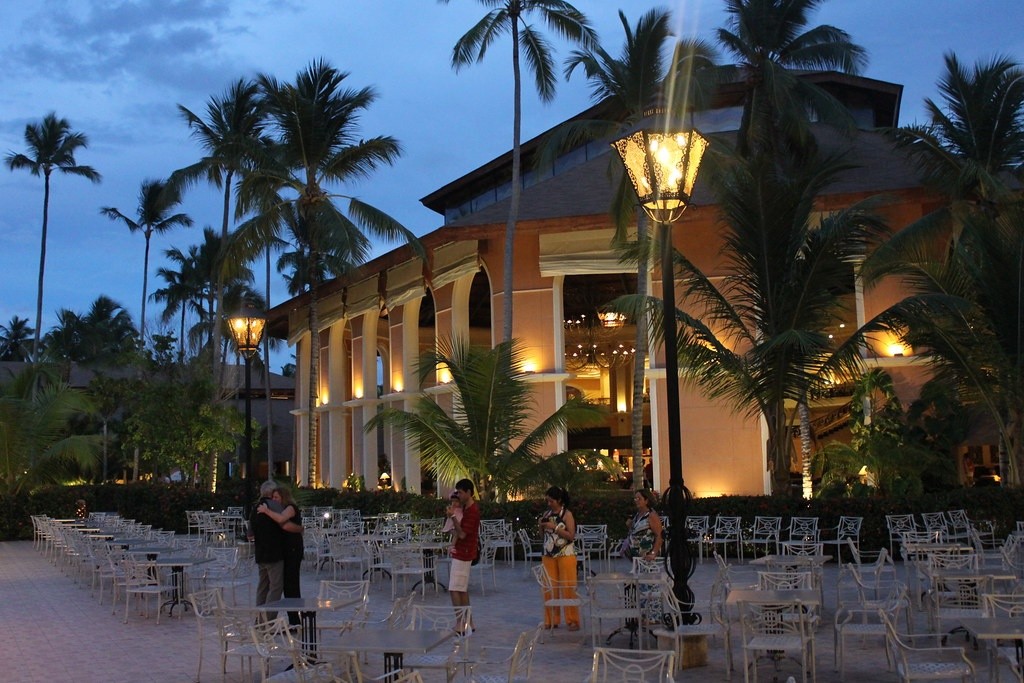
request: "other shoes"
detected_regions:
[541,623,558,629]
[569,623,578,631]
[281,627,298,634]
[447,546,456,554]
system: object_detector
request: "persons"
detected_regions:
[247,481,305,631]
[257,486,304,634]
[445,479,480,636]
[443,491,463,554]
[625,488,663,625]
[537,486,581,632]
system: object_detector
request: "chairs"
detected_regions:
[26,501,1024,683]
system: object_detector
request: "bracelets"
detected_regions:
[555,526,559,534]
[450,514,456,519]
[650,551,656,556]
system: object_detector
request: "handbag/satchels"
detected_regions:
[619,537,632,558]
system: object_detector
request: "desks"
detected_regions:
[307,528,344,571]
[254,595,364,674]
[750,553,833,608]
[138,556,218,617]
[104,538,156,550]
[355,533,401,580]
[89,531,128,567]
[899,541,974,601]
[724,587,820,677]
[52,518,83,523]
[75,526,116,533]
[62,522,93,527]
[217,514,243,535]
[590,568,668,650]
[574,532,599,577]
[121,543,189,598]
[321,628,457,683]
[392,536,453,595]
[960,617,1024,683]
[920,564,1017,651]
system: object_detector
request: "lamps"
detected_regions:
[562,313,636,374]
[380,472,390,480]
[595,306,626,327]
